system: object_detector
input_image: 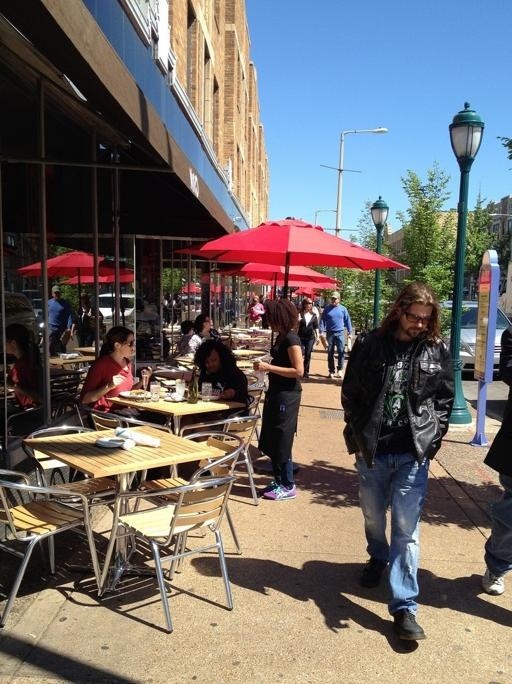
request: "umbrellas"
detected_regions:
[210,262,339,351]
[62,276,135,327]
[174,218,411,296]
[18,249,134,318]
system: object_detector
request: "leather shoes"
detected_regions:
[391,610,427,641]
[360,555,388,587]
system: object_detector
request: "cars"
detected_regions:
[96,292,134,323]
[439,300,512,374]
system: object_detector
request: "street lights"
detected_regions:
[449,101,485,425]
[335,128,388,235]
[314,209,338,226]
[369,196,389,328]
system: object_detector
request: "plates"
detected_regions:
[198,392,222,400]
[96,438,125,447]
[119,390,151,399]
[165,397,184,402]
[8,389,15,392]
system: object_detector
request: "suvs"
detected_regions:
[1,291,39,349]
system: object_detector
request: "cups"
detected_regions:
[202,383,212,402]
[176,379,185,397]
[150,382,160,402]
[142,372,150,402]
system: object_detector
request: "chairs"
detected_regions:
[7,401,46,439]
[180,414,262,505]
[74,403,89,428]
[50,378,79,419]
[223,388,263,456]
[22,426,117,575]
[130,431,246,580]
[84,406,177,479]
[0,469,101,629]
[99,476,238,630]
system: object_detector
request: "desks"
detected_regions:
[231,350,266,356]
[172,357,254,372]
[49,356,96,370]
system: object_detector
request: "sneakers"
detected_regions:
[260,480,279,496]
[482,567,506,596]
[264,485,297,500]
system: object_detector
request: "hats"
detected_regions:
[330,291,341,301]
[52,285,63,293]
[253,295,260,302]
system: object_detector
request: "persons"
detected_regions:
[79,325,153,430]
[162,292,185,323]
[70,291,104,353]
[180,339,250,441]
[340,280,457,641]
[248,294,270,329]
[319,291,353,379]
[5,322,46,430]
[252,299,304,500]
[299,298,320,378]
[47,285,75,357]
[481,325,512,596]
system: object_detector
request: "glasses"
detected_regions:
[121,340,136,347]
[403,310,433,325]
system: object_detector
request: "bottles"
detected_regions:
[187,371,198,403]
